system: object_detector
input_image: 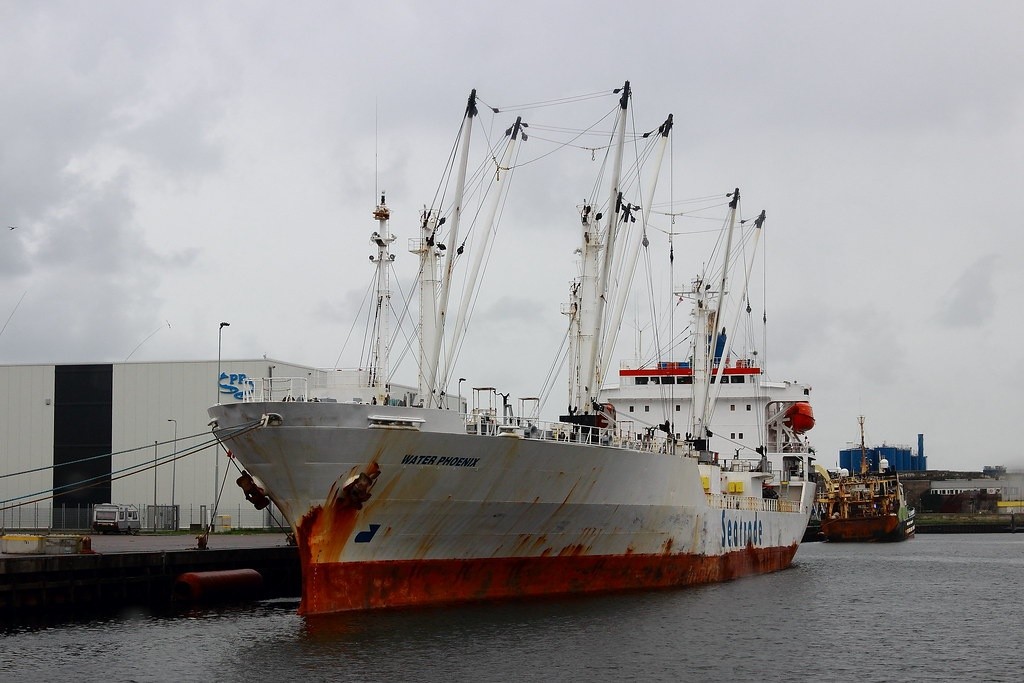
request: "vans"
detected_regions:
[92,502,142,535]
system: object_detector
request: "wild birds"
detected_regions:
[9,226,18,230]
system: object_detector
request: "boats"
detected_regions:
[784,403,816,433]
[815,416,917,543]
[206,78,818,617]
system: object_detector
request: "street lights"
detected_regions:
[214,321,230,510]
[168,419,177,530]
